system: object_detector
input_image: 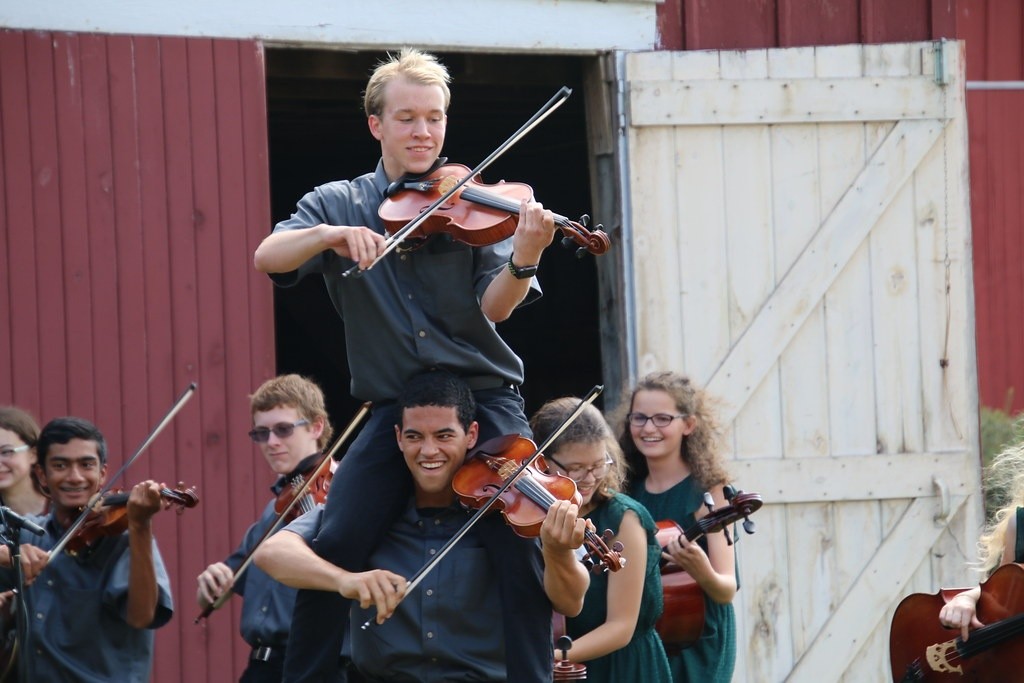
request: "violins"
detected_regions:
[63,480,200,551]
[451,432,627,572]
[378,163,611,256]
[273,454,342,521]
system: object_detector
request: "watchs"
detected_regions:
[507,251,539,280]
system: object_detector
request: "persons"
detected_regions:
[196,373,352,683]
[253,366,591,683]
[529,397,674,683]
[253,46,555,683]
[0,406,175,683]
[938,443,1024,642]
[618,369,742,683]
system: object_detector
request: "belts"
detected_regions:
[469,376,517,390]
[249,647,286,665]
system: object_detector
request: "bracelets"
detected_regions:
[952,594,975,600]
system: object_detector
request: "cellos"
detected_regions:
[644,483,763,651]
[889,562,1024,683]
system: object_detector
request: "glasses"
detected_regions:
[546,453,613,483]
[0,445,30,460]
[247,420,308,441]
[627,413,686,427]
